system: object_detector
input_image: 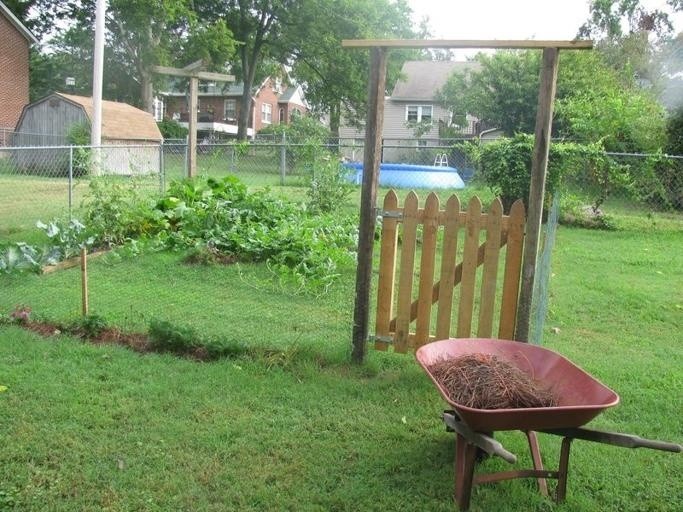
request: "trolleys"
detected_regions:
[414,337,681,509]
